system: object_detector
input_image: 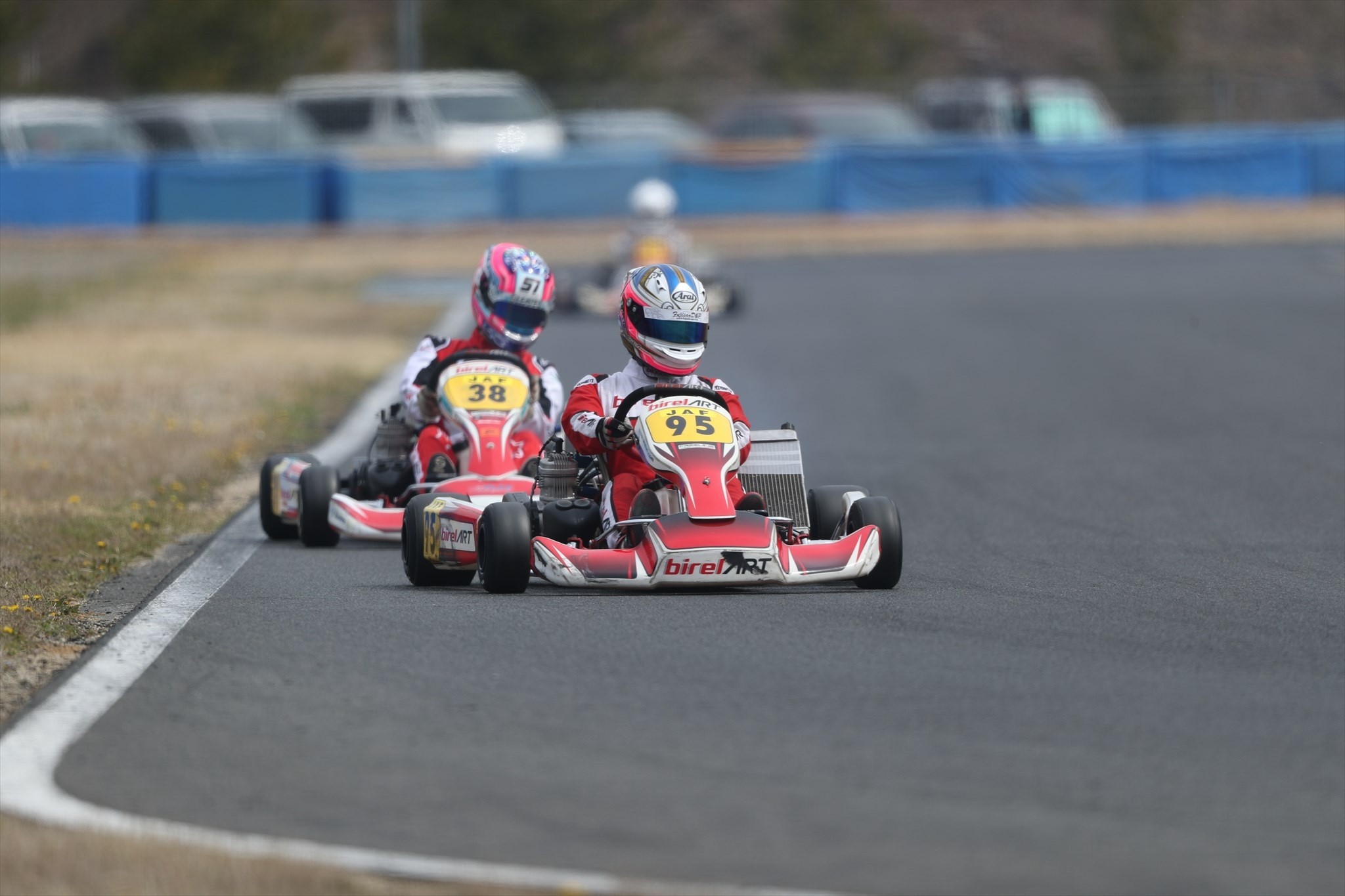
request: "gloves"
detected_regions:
[528,374,540,403]
[597,416,637,451]
[417,387,442,418]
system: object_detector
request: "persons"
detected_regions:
[401,243,566,494]
[561,264,753,550]
[590,178,696,288]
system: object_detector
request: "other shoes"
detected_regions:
[734,492,766,510]
[425,453,457,493]
[625,488,661,549]
[518,457,542,478]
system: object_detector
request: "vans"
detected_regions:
[276,69,563,160]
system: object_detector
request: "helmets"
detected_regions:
[618,264,710,377]
[472,242,557,352]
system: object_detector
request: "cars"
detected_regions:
[0,95,123,158]
[562,105,699,146]
[914,76,1122,143]
[117,94,290,159]
[706,91,929,141]
